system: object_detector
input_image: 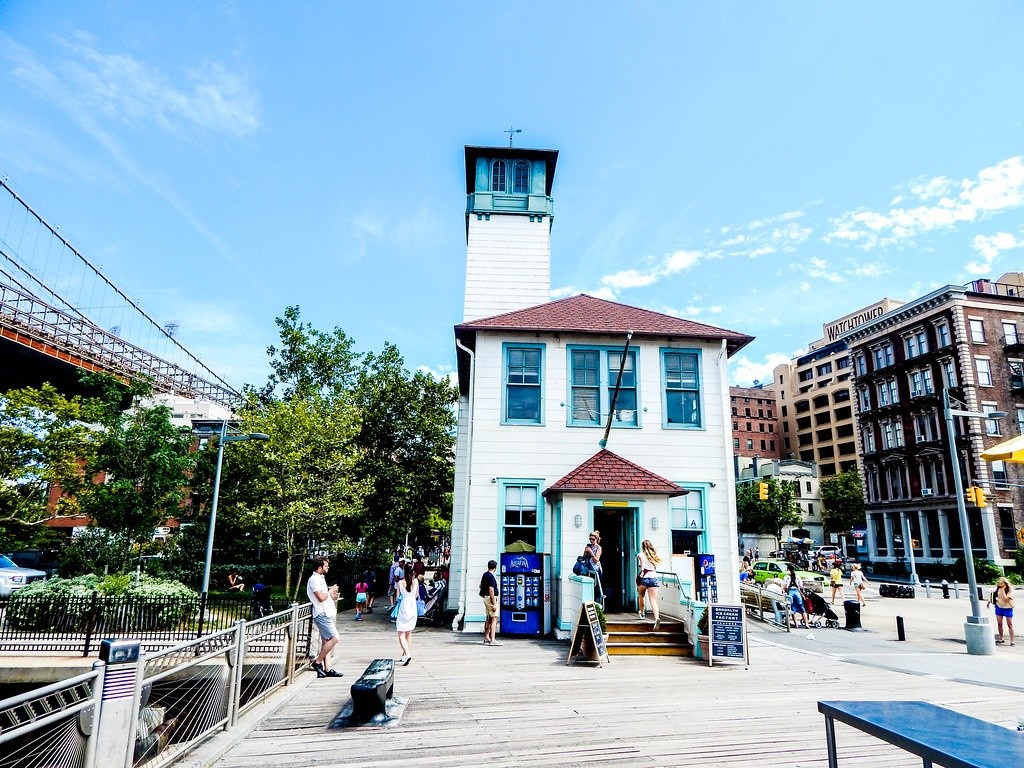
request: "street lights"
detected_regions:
[195,432,270,655]
[944,402,1007,619]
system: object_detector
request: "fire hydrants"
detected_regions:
[942,579,951,599]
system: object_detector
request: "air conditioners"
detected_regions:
[917,434,927,443]
[920,488,932,496]
[881,401,887,406]
[863,406,870,412]
[911,390,921,397]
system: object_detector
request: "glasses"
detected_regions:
[589,538,596,541]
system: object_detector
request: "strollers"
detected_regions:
[799,586,840,629]
[389,578,448,622]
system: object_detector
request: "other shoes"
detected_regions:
[402,656,412,666]
[391,619,396,622]
[1011,642,1015,645]
[489,640,503,646]
[995,638,1005,643]
[400,656,406,661]
[483,639,491,645]
[388,606,394,612]
[653,618,660,631]
[639,610,645,618]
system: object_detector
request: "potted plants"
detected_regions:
[580,602,610,661]
[698,606,722,661]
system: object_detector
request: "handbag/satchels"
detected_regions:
[860,584,865,589]
[391,595,402,618]
[991,588,999,604]
[830,581,835,586]
[479,590,485,598]
[805,597,813,612]
[636,576,642,586]
[416,600,425,616]
[573,556,589,576]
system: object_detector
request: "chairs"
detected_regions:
[417,583,446,623]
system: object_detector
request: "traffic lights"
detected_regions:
[966,488,972,502]
[982,491,988,508]
[762,483,768,500]
[1017,529,1024,543]
[913,540,920,548]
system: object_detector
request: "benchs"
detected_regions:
[328,659,410,727]
[818,700,1024,768]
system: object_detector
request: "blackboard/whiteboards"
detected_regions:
[569,601,608,660]
[708,603,748,660]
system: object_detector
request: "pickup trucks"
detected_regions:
[0,554,47,598]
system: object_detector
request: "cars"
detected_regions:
[152,534,175,542]
[768,549,810,572]
[5,553,13,559]
[129,555,169,566]
[752,560,830,590]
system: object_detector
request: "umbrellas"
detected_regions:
[779,536,801,549]
[979,433,1024,465]
[795,537,819,557]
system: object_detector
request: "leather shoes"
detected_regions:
[323,669,343,677]
[312,660,326,676]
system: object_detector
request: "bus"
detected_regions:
[179,521,195,539]
[71,526,123,545]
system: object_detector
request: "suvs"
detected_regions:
[812,545,842,559]
[12,551,55,569]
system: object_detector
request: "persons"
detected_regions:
[986,577,1015,645]
[781,565,810,629]
[850,564,871,607]
[829,562,846,606]
[637,540,662,631]
[252,576,268,618]
[352,542,450,622]
[766,579,791,625]
[584,530,603,612]
[227,568,245,592]
[306,556,343,678]
[740,546,761,597]
[394,562,419,666]
[786,549,828,572]
[479,560,503,646]
